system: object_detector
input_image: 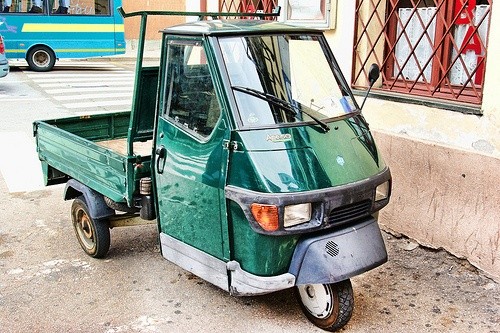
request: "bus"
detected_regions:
[0,0,126,73]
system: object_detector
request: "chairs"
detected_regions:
[207,88,245,128]
[0,0,72,15]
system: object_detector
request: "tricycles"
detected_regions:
[31,6,393,333]
